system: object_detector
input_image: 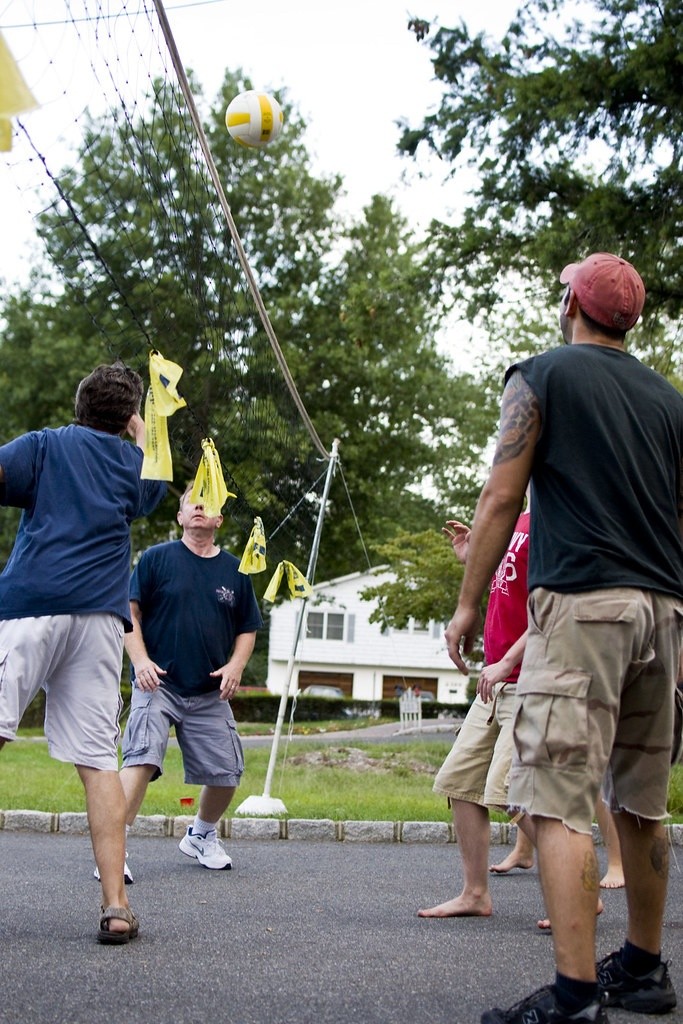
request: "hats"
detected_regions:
[559,252,645,330]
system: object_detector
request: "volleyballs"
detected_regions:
[225,89,284,149]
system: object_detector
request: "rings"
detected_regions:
[232,686,235,688]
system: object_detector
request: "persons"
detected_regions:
[418,483,603,930]
[446,253,683,1024]
[94,480,265,884]
[488,782,625,888]
[0,364,166,945]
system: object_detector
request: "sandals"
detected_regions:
[97,903,139,944]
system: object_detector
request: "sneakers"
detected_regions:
[595,947,678,1016]
[93,850,133,884]
[480,985,610,1024]
[178,825,232,870]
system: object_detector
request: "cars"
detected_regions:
[302,684,351,716]
[405,690,434,701]
